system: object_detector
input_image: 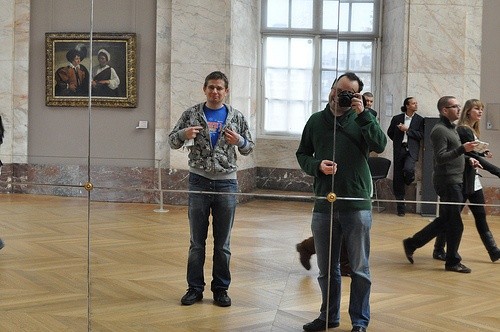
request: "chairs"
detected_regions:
[363,157,391,213]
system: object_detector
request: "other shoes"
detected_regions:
[432,251,447,261]
[212,290,232,306]
[396,201,406,216]
[181,289,204,304]
[351,326,367,332]
[402,237,415,264]
[446,261,472,273]
[490,248,500,262]
[303,319,341,332]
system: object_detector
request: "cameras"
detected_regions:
[337,90,358,107]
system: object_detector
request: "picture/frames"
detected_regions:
[44,30,137,109]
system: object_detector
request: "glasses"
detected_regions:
[332,88,344,94]
[446,105,462,109]
[206,85,226,91]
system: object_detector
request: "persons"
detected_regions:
[431,99,500,262]
[403,95,478,274]
[296,72,387,332]
[168,71,255,307]
[296,237,350,277]
[362,92,377,117]
[387,97,424,216]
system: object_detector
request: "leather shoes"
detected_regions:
[338,268,352,276]
[297,243,311,270]
